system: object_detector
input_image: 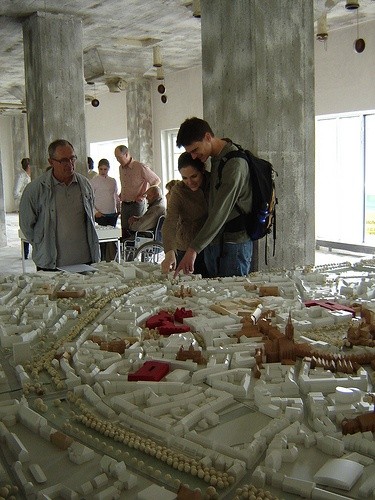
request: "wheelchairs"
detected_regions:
[113,214,166,264]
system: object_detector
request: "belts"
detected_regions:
[121,201,145,207]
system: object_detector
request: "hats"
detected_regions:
[143,185,162,195]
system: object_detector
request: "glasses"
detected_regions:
[49,155,80,165]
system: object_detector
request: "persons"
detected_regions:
[164,180,180,213]
[13,158,31,260]
[85,158,96,177]
[174,116,252,278]
[115,145,161,247]
[19,140,101,271]
[160,152,209,275]
[124,186,164,247]
[90,158,120,261]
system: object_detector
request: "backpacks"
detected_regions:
[215,143,280,240]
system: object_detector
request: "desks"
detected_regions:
[18,228,122,263]
